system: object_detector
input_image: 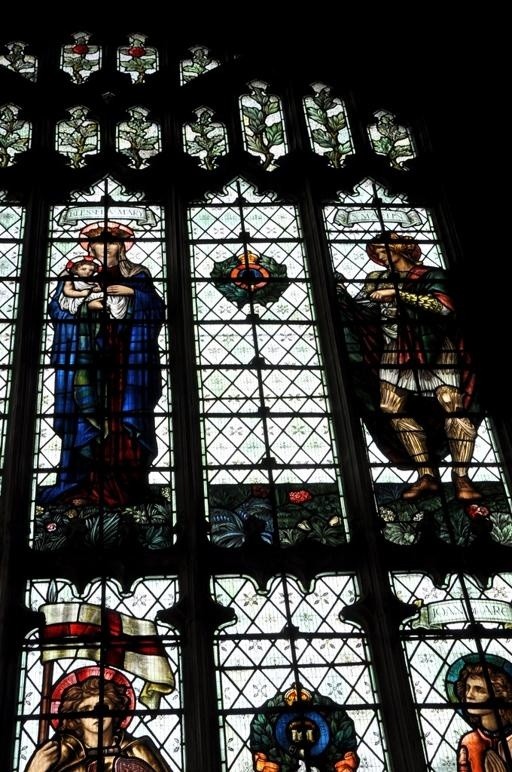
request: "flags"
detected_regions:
[40,601,179,714]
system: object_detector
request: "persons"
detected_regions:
[49,213,163,507]
[61,256,127,318]
[445,656,512,771]
[342,227,490,502]
[26,665,171,772]
